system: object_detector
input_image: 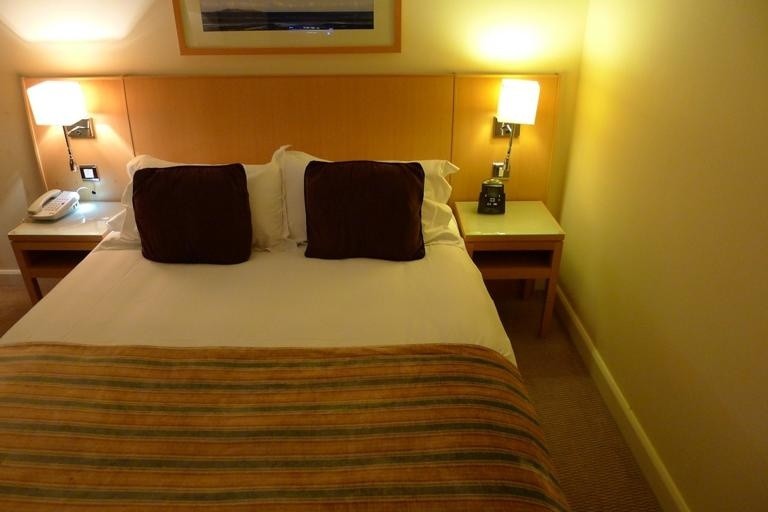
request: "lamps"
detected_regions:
[27,76,90,172]
[491,78,541,178]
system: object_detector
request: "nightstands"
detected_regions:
[7,200,122,307]
[454,200,566,339]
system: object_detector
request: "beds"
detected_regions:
[0,75,569,511]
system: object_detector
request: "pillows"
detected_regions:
[303,159,426,263]
[284,148,460,248]
[123,143,293,254]
[130,163,253,264]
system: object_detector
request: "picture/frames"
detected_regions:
[172,0,402,56]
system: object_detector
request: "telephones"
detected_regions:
[27,189,80,221]
